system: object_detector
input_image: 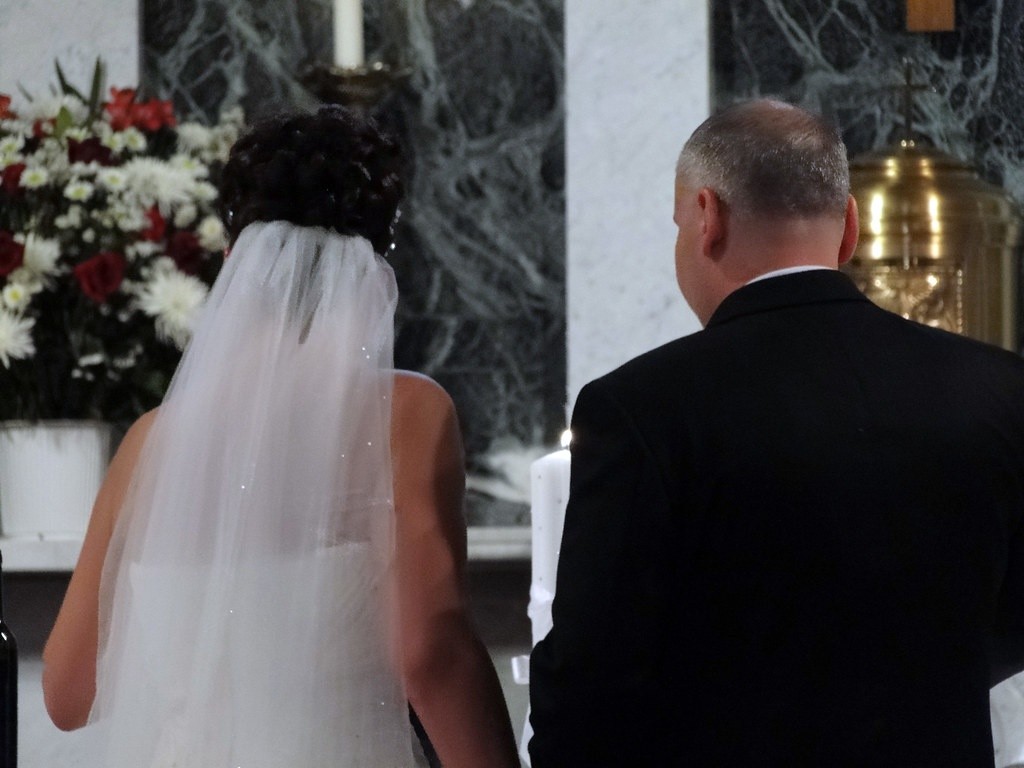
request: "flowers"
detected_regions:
[1,55,245,425]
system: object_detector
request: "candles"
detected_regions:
[527,426,572,648]
[332,0,365,68]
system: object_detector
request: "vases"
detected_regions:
[1,419,126,543]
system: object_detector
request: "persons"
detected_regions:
[527,95,1024,768]
[43,107,521,768]
